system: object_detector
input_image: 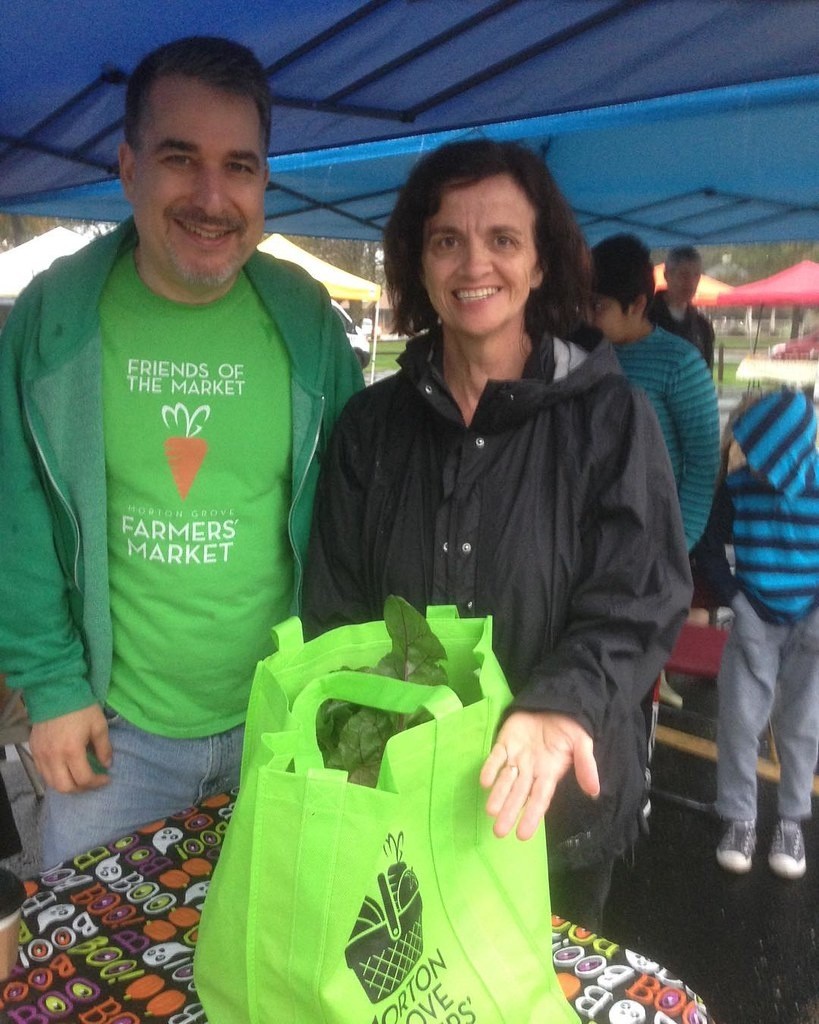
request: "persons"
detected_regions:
[0,36,367,936]
[699,391,819,880]
[304,139,692,934]
[650,246,717,370]
[582,232,724,548]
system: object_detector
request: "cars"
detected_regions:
[331,298,370,370]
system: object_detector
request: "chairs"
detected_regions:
[642,560,780,824]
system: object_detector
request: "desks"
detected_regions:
[0,787,717,1024]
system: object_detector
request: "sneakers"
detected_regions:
[769,816,808,878]
[715,822,758,871]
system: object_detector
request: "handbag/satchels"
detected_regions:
[190,601,588,1024]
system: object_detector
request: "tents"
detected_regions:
[254,234,381,385]
[713,260,818,379]
[650,264,751,359]
[2,226,89,309]
